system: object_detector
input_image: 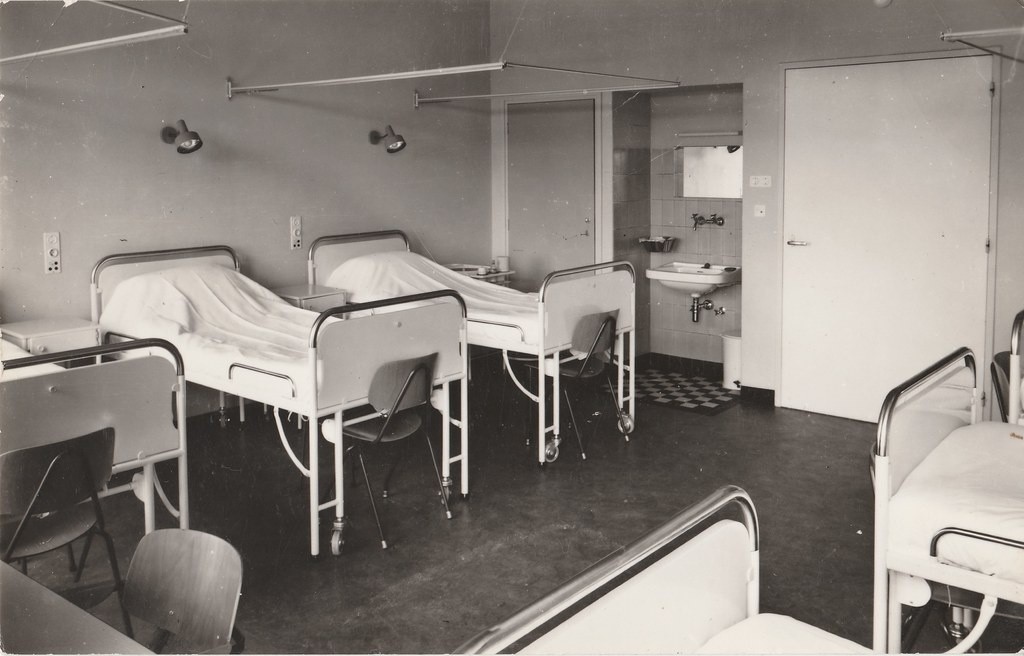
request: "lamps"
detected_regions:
[368,124,406,153]
[161,119,203,154]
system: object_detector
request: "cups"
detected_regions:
[478,266,490,275]
[496,256,509,272]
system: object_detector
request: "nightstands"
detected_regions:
[444,264,515,378]
[264,288,346,434]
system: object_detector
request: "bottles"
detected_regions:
[490,260,497,273]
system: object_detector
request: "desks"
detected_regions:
[0,559,157,654]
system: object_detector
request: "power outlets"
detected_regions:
[44,232,61,275]
[290,216,302,249]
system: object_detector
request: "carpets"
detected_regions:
[602,364,752,418]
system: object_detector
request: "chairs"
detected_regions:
[298,352,452,549]
[523,309,629,458]
[0,426,135,640]
[123,527,246,654]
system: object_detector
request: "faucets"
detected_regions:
[690,213,706,231]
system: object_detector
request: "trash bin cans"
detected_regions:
[719,329,741,390]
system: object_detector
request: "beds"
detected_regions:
[91,244,470,565]
[455,311,1024,656]
[308,230,637,465]
[1,336,189,531]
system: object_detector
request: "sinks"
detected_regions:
[645,261,742,295]
